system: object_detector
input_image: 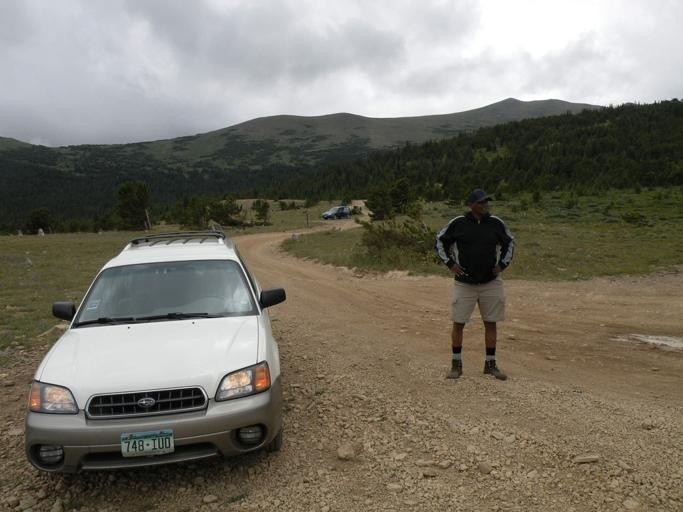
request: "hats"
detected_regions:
[467,187,493,203]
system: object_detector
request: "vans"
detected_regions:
[321,205,352,220]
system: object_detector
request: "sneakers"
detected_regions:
[445,358,463,379]
[482,358,508,380]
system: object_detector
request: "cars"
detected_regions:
[22,228,287,488]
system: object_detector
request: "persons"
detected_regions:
[435,188,516,381]
